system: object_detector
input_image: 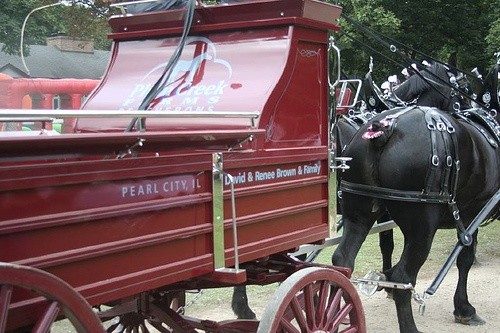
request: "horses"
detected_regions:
[336,51,500,333]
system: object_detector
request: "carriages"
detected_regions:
[0,0,500,333]
